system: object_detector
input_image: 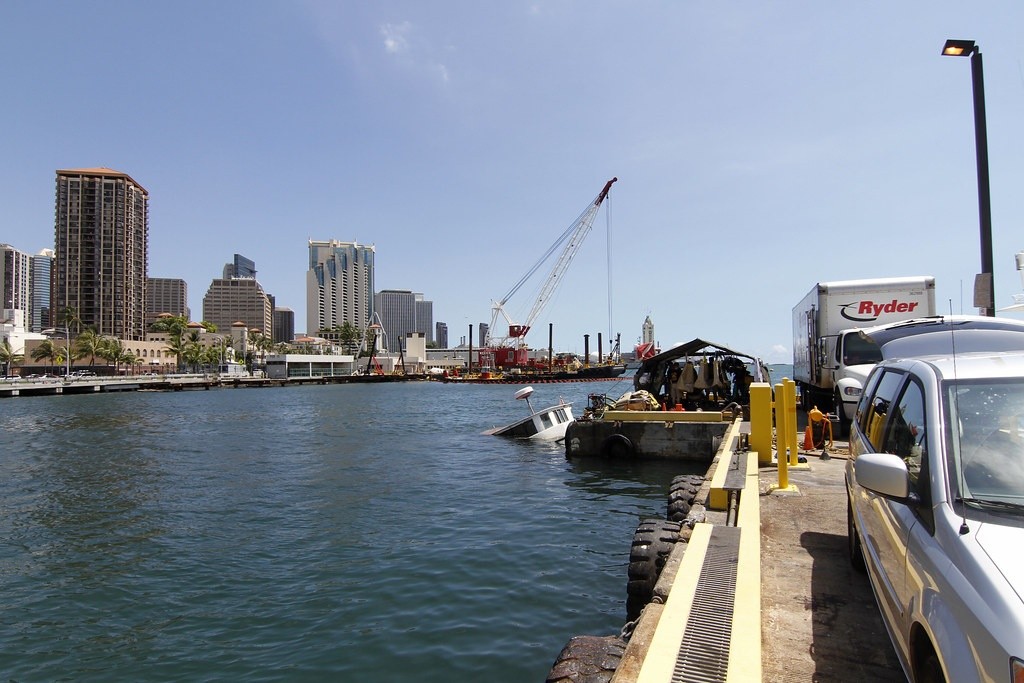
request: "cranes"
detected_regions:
[483,176,619,373]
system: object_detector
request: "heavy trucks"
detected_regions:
[792,276,938,431]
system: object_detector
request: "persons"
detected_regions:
[663,354,757,413]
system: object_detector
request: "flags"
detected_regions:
[636,341,655,361]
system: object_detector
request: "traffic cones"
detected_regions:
[801,426,820,451]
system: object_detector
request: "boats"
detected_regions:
[480,385,577,447]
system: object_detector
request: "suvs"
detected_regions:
[844,313,1024,683]
